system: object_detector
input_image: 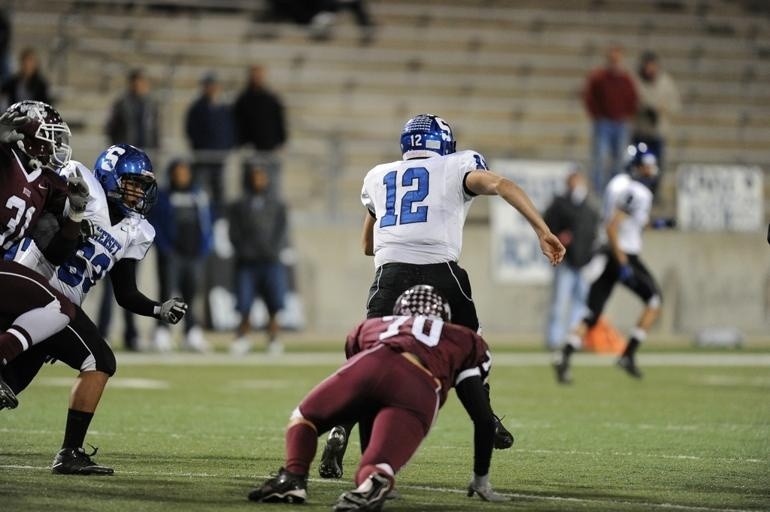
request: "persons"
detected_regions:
[247,282,511,510]
[178,70,234,204]
[150,158,213,352]
[227,157,288,356]
[98,278,140,353]
[7,46,52,104]
[582,47,641,194]
[543,173,602,350]
[0,99,89,410]
[102,67,162,169]
[1,146,189,476]
[318,114,568,479]
[629,45,684,210]
[229,58,287,200]
[553,138,676,385]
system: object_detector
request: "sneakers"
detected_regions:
[618,356,641,378]
[187,326,204,350]
[556,361,567,382]
[147,327,172,352]
[333,471,393,512]
[319,425,347,479]
[50,444,113,475]
[0,379,18,410]
[248,466,306,504]
[484,414,515,449]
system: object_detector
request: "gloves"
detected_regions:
[0,111,31,143]
[66,167,89,212]
[467,474,511,502]
[159,296,188,324]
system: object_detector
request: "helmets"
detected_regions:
[400,113,456,160]
[94,143,158,221]
[624,140,661,185]
[391,284,452,323]
[7,100,72,175]
